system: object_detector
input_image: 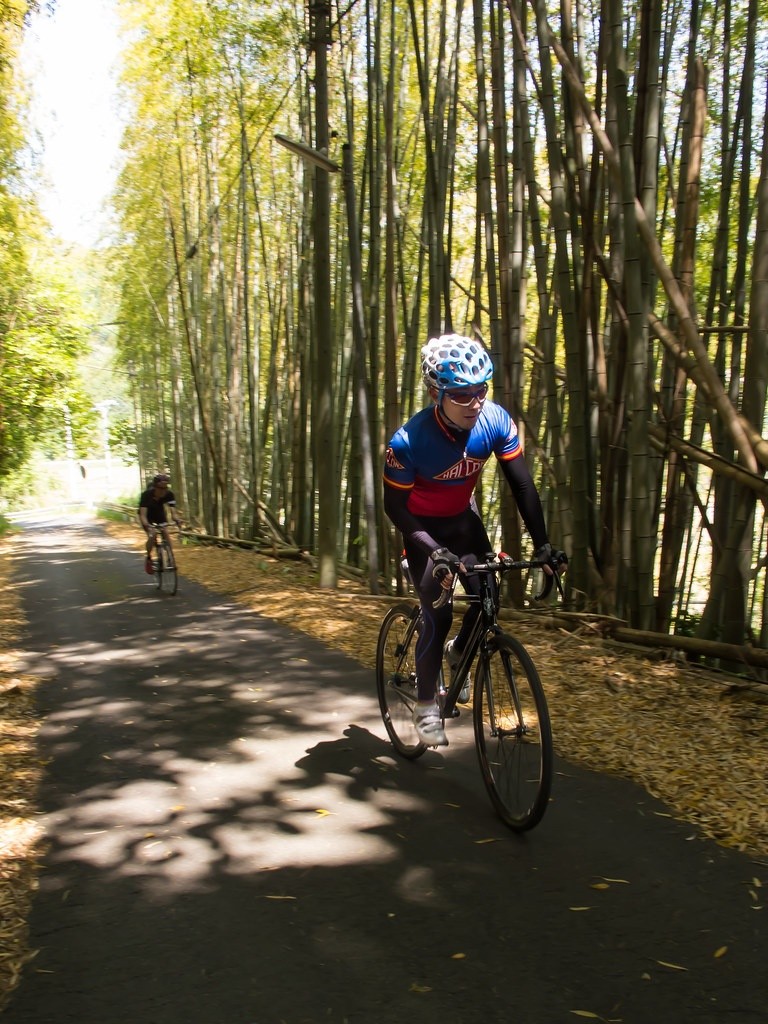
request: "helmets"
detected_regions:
[154,475,170,488]
[421,334,494,389]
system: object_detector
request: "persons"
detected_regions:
[380,331,569,749]
[140,475,185,575]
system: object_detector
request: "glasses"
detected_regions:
[431,381,489,407]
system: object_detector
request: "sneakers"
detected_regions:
[444,639,471,703]
[412,701,449,747]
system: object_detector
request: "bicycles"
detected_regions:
[143,521,184,596]
[375,541,567,835]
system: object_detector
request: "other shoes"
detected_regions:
[145,559,154,574]
